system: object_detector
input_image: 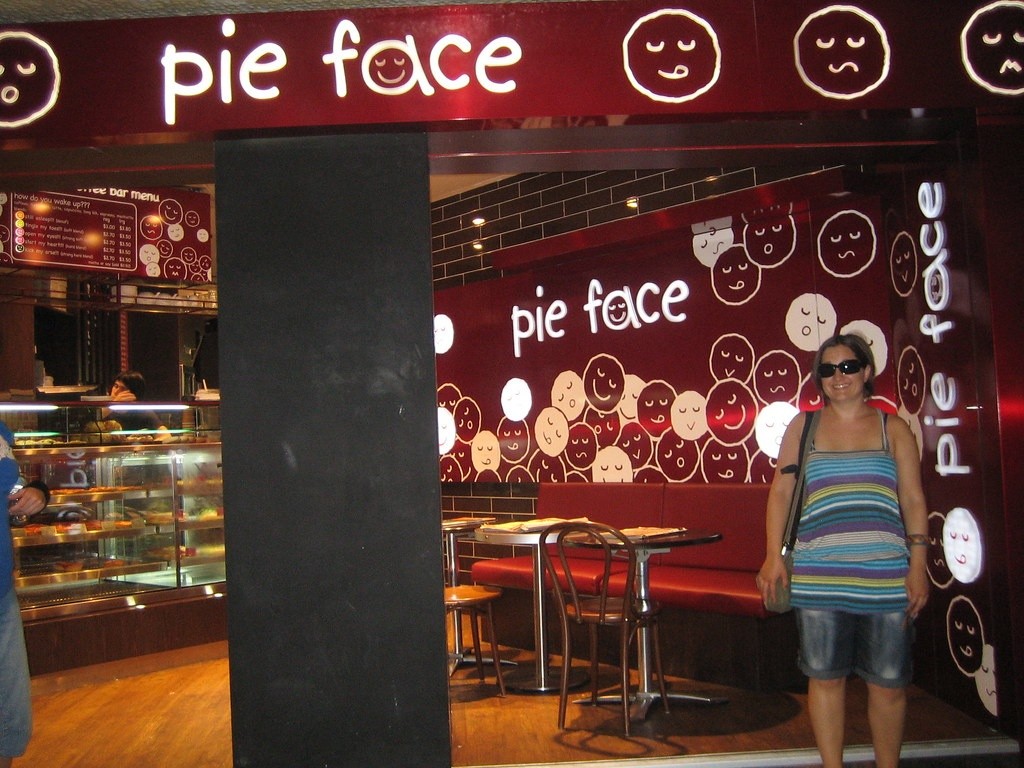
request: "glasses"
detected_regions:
[817,359,865,378]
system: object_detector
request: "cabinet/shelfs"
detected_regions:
[0,401,229,677]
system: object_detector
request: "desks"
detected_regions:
[441,517,519,676]
[564,528,725,722]
[474,522,610,691]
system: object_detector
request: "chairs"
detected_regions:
[540,522,671,739]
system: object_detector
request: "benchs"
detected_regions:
[470,482,810,694]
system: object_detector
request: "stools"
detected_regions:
[443,585,509,697]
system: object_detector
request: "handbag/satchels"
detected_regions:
[761,547,791,614]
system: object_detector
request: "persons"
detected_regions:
[0,419,51,768]
[757,334,930,768]
[79,370,172,442]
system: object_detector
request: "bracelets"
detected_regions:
[22,480,51,503]
[907,534,930,546]
